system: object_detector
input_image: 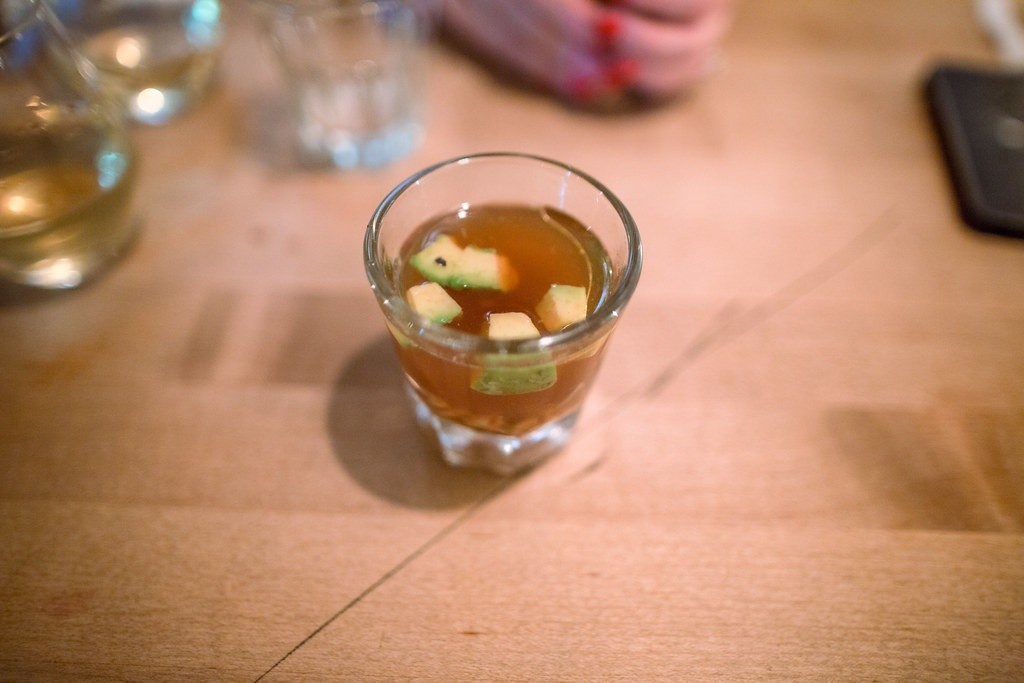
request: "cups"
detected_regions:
[257,1,432,178]
[77,1,217,132]
[0,0,140,292]
[364,152,643,477]
